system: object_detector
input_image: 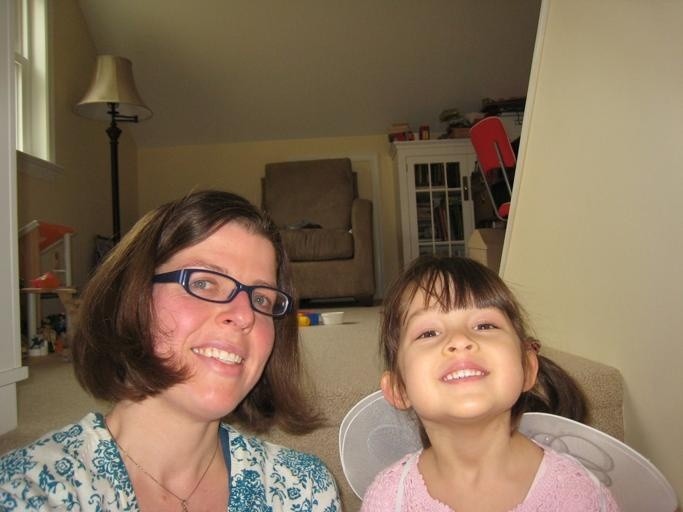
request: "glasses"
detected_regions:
[152,268,294,318]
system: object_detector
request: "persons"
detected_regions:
[358,255,620,511]
[0,188,345,511]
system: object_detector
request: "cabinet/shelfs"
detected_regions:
[391,143,478,271]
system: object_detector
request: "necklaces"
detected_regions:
[104,415,219,512]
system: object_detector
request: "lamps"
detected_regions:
[74,55,153,246]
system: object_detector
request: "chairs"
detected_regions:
[260,157,375,306]
[226,325,625,512]
[470,117,517,221]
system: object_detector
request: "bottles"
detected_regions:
[419,125,430,140]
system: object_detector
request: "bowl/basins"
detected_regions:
[321,312,344,324]
